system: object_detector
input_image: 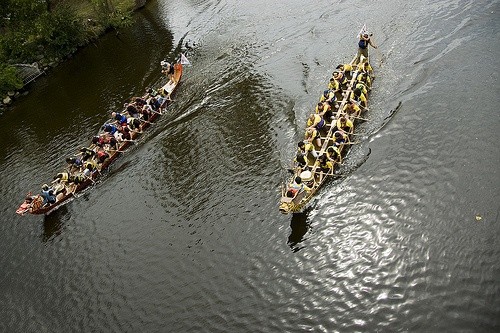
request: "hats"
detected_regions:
[160,61,165,66]
[354,88,361,97]
[324,90,330,94]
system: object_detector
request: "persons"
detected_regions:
[356,31,378,65]
[328,78,345,98]
[315,154,334,178]
[355,83,369,100]
[304,128,323,148]
[325,146,342,166]
[160,60,177,85]
[357,72,373,91]
[349,88,368,110]
[296,141,318,159]
[25,196,33,204]
[332,131,351,148]
[292,154,310,170]
[40,86,171,210]
[306,113,329,135]
[289,176,316,192]
[331,71,349,88]
[320,90,339,109]
[332,116,354,137]
[358,62,375,78]
[342,101,362,120]
[337,64,354,78]
[315,101,335,120]
